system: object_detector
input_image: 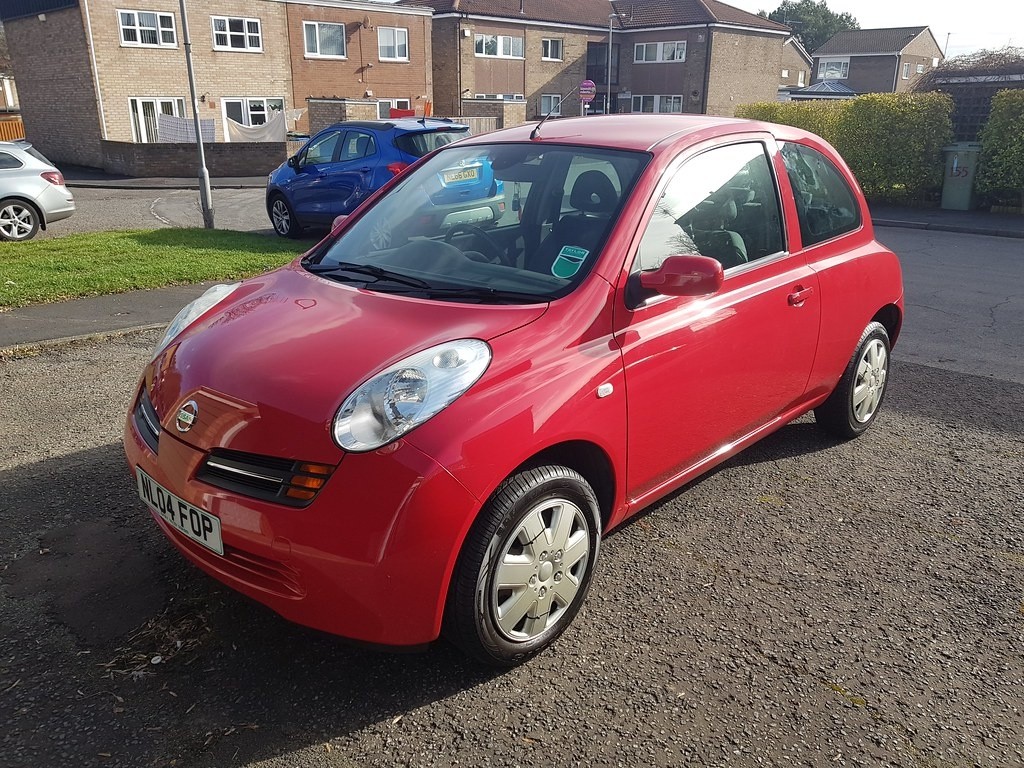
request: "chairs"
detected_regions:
[525,170,618,283]
[435,134,451,150]
[350,137,375,159]
[682,183,813,270]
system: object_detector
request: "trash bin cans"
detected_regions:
[939,142,984,210]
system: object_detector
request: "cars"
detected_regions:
[0,136,76,242]
[265,116,506,252]
[122,113,903,672]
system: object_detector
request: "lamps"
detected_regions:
[418,96,420,98]
[466,89,470,91]
